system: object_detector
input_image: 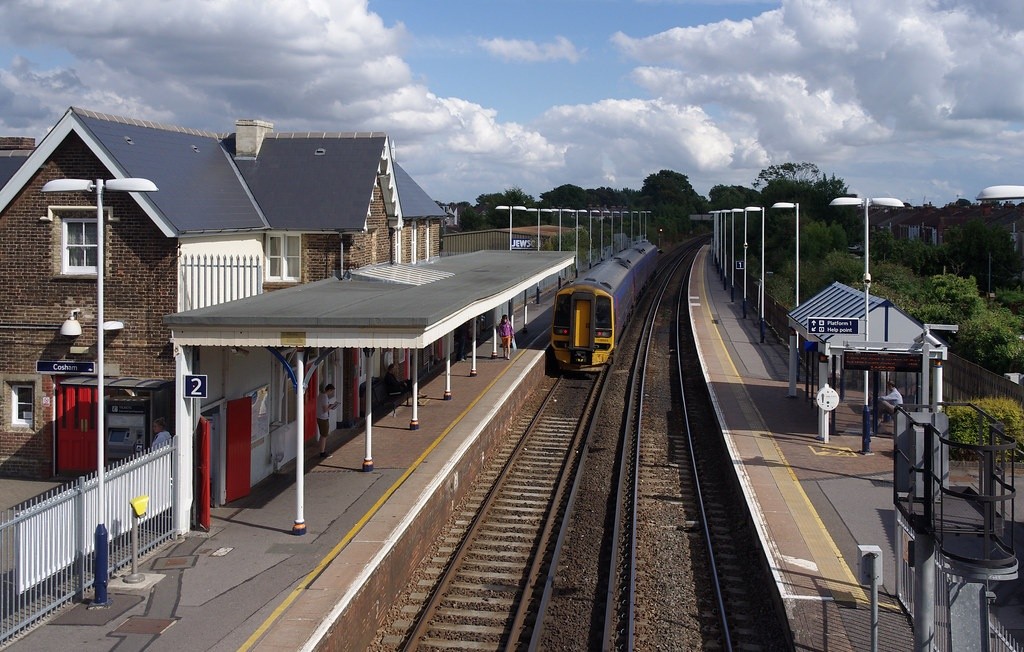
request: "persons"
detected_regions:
[151,417,171,452]
[498,314,514,360]
[316,384,337,459]
[878,381,903,426]
[459,324,469,361]
[385,364,428,406]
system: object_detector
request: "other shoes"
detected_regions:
[320,452,332,458]
[504,355,507,358]
[870,430,874,436]
[507,357,510,360]
[461,358,465,362]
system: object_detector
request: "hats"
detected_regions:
[388,364,394,370]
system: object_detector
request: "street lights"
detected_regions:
[601,210,611,261]
[497,206,527,250]
[732,208,749,317]
[713,210,720,273]
[745,206,766,343]
[551,207,570,250]
[526,208,553,252]
[611,210,620,256]
[41,178,160,606]
[644,210,652,240]
[590,209,600,269]
[721,209,736,302]
[830,197,905,455]
[772,202,800,384]
[709,210,716,265]
[632,210,639,244]
[620,210,629,251]
[638,211,646,242]
[566,209,587,269]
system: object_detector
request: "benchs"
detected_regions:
[373,383,412,418]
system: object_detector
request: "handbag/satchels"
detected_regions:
[512,338,517,350]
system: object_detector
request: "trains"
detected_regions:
[551,240,664,373]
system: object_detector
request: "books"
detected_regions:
[331,401,341,410]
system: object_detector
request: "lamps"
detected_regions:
[59,310,81,335]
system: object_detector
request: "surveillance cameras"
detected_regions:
[60,320,82,340]
[925,334,942,348]
[913,333,923,343]
[864,273,871,283]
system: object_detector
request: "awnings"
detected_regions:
[167,251,577,350]
